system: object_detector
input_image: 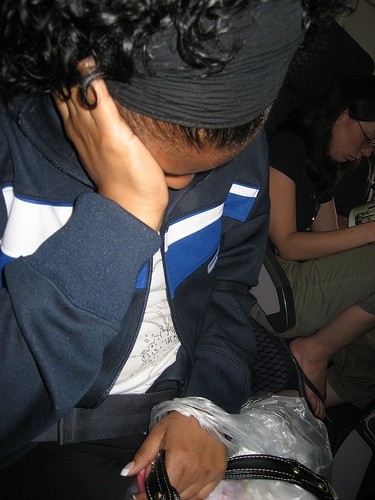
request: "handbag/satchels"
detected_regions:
[152,390,331,500]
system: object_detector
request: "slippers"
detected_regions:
[281,337,327,419]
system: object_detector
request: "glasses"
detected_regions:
[357,120,375,153]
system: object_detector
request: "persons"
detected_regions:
[0,0,361,500]
[250,74,375,421]
